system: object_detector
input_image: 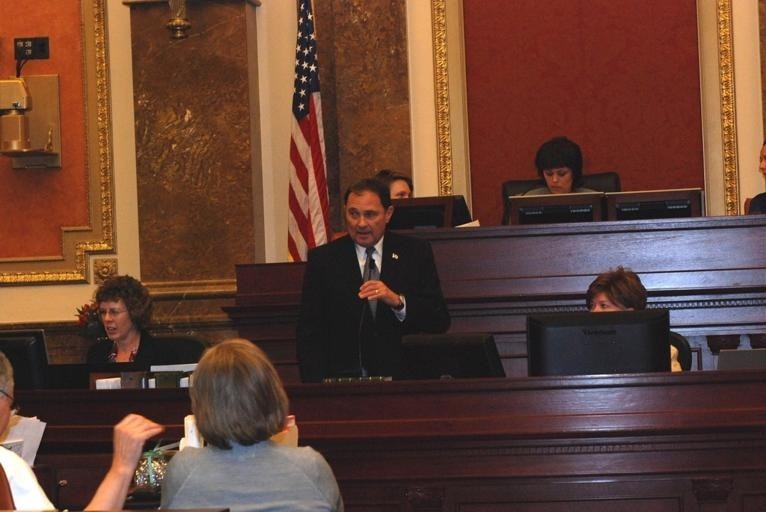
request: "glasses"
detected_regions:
[0,388,25,417]
[96,307,129,318]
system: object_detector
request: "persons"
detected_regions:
[0,348,164,512]
[295,178,451,379]
[584,267,681,373]
[376,168,412,199]
[514,137,599,196]
[90,274,179,366]
[161,337,344,511]
[749,142,765,214]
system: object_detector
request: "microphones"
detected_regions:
[356,258,376,378]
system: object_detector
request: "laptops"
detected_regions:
[401,334,506,380]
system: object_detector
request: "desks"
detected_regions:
[14,371,765,512]
[222,213,766,381]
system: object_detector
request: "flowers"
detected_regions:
[74,302,101,331]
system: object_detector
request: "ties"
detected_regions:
[359,248,383,316]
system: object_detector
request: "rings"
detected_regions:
[375,289,379,295]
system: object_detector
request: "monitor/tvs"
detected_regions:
[509,191,606,226]
[388,195,473,228]
[527,307,671,377]
[606,188,705,221]
[0,329,51,389]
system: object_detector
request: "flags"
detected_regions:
[286,1,331,261]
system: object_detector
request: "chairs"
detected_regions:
[89,336,210,362]
[669,331,693,370]
[502,172,619,225]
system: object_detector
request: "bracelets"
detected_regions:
[395,293,405,310]
